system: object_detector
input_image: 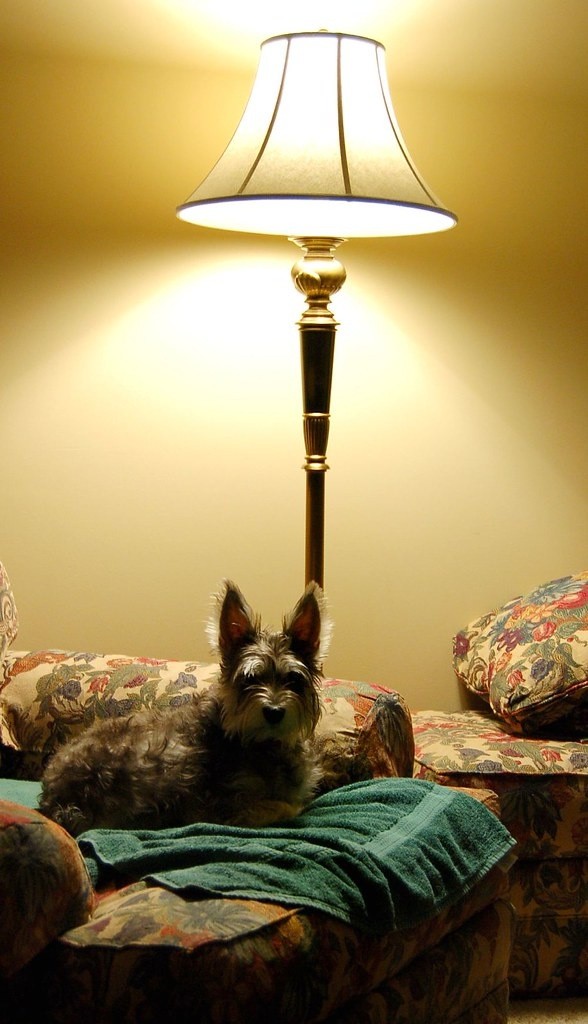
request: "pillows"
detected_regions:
[453,573,588,734]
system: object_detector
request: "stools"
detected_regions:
[410,710,588,1000]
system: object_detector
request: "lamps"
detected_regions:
[177,32,460,612]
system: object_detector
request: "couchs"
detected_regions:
[0,649,512,1024]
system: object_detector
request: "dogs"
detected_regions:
[35,578,331,834]
